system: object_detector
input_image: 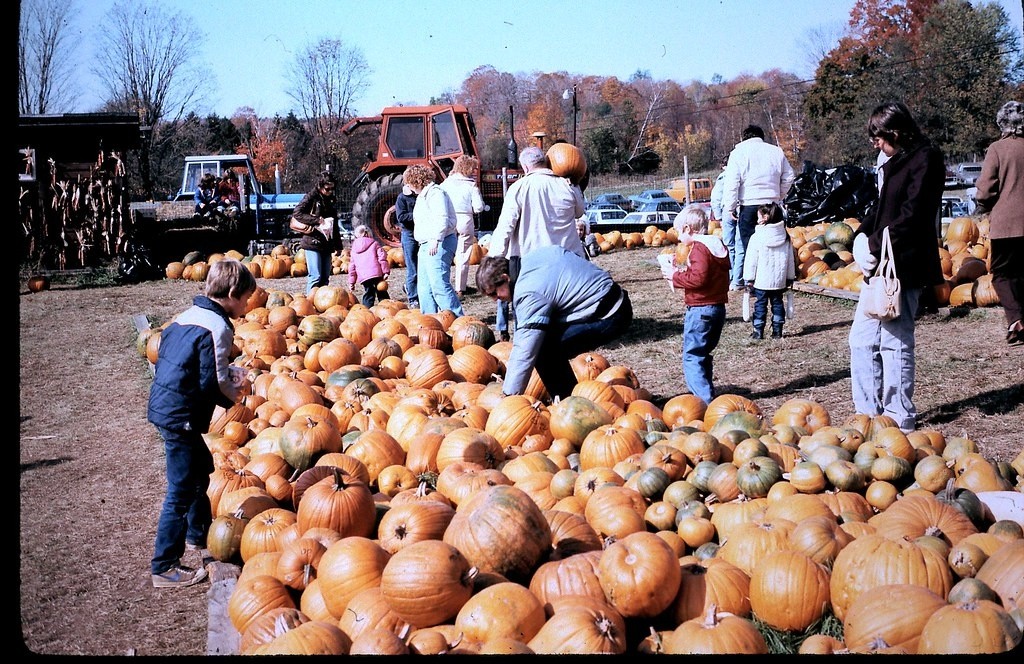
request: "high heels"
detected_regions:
[1007,321,1024,344]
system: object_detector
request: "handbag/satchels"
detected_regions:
[290,194,316,233]
[860,229,902,323]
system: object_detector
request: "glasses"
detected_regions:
[322,185,335,192]
[870,137,886,146]
[487,283,498,297]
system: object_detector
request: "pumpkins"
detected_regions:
[28,275,49,292]
[676,217,1001,309]
[593,226,680,252]
[545,142,587,182]
[137,281,1023,655]
[165,236,488,280]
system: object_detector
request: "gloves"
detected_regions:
[852,233,878,277]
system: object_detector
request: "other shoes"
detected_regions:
[771,330,781,339]
[501,332,509,342]
[229,210,236,218]
[752,329,762,340]
[456,291,463,301]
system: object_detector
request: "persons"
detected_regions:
[192,173,220,219]
[395,139,590,341]
[743,201,796,340]
[846,100,945,434]
[218,168,246,219]
[149,260,257,587]
[975,101,1024,344]
[711,124,796,291]
[661,206,732,404]
[293,171,343,295]
[348,225,391,310]
[474,245,633,404]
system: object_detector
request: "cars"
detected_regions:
[590,193,633,210]
[943,160,958,186]
[957,162,983,190]
[627,189,677,208]
[590,204,623,210]
[620,211,679,223]
[638,201,684,214]
[585,209,629,225]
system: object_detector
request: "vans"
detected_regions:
[664,177,713,204]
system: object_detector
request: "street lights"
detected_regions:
[562,83,578,147]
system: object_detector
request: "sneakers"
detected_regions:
[151,568,207,589]
[186,542,202,552]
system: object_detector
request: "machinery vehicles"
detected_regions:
[117,154,311,259]
[341,104,528,248]
